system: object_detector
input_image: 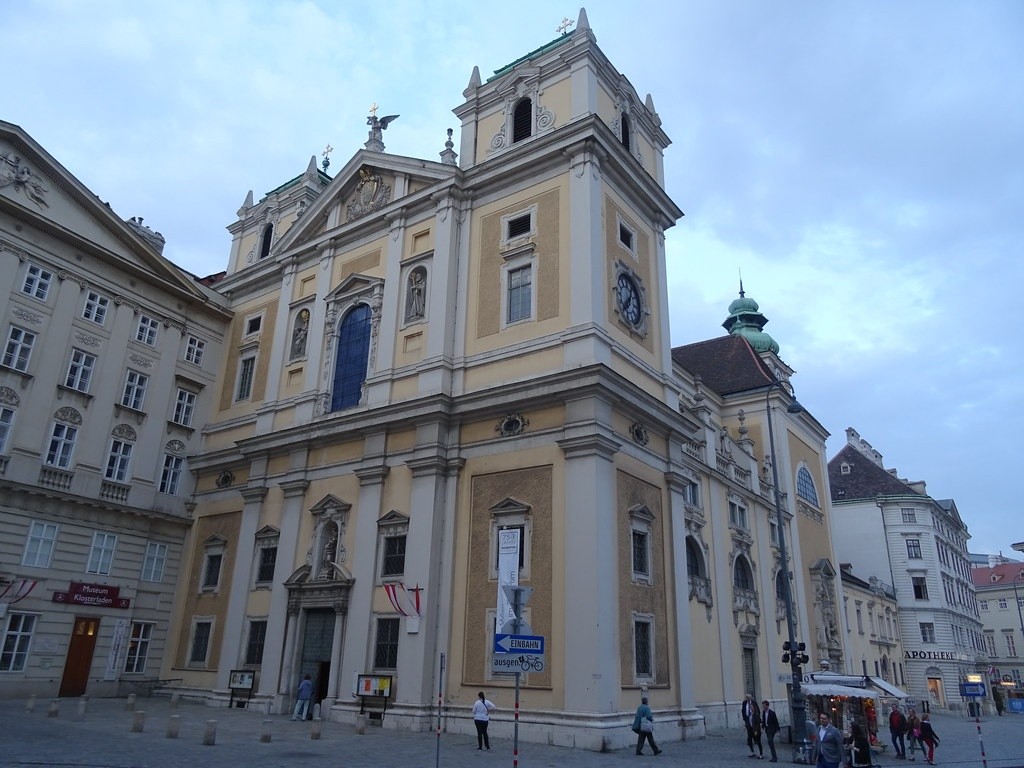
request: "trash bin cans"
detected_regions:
[969,701,981,718]
[779,725,791,744]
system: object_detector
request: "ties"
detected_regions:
[749,701,750,716]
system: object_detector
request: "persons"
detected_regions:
[290,674,313,721]
[366,115,400,141]
[408,273,425,318]
[811,712,847,768]
[907,708,927,761]
[319,531,338,580]
[761,454,776,486]
[295,312,308,357]
[992,686,1004,717]
[920,714,940,765]
[741,693,764,759]
[819,581,838,642]
[471,691,496,751]
[761,700,781,762]
[15,166,49,210]
[720,425,737,462]
[632,697,663,756]
[889,704,907,759]
[843,721,871,768]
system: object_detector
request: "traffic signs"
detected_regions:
[494,634,544,655]
[960,683,986,696]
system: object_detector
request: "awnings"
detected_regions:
[869,676,912,700]
[796,684,880,701]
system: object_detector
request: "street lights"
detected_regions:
[766,380,815,765]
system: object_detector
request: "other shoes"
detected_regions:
[636,752,644,755]
[654,750,662,756]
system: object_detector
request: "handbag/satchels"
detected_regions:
[640,716,654,733]
[907,729,912,740]
